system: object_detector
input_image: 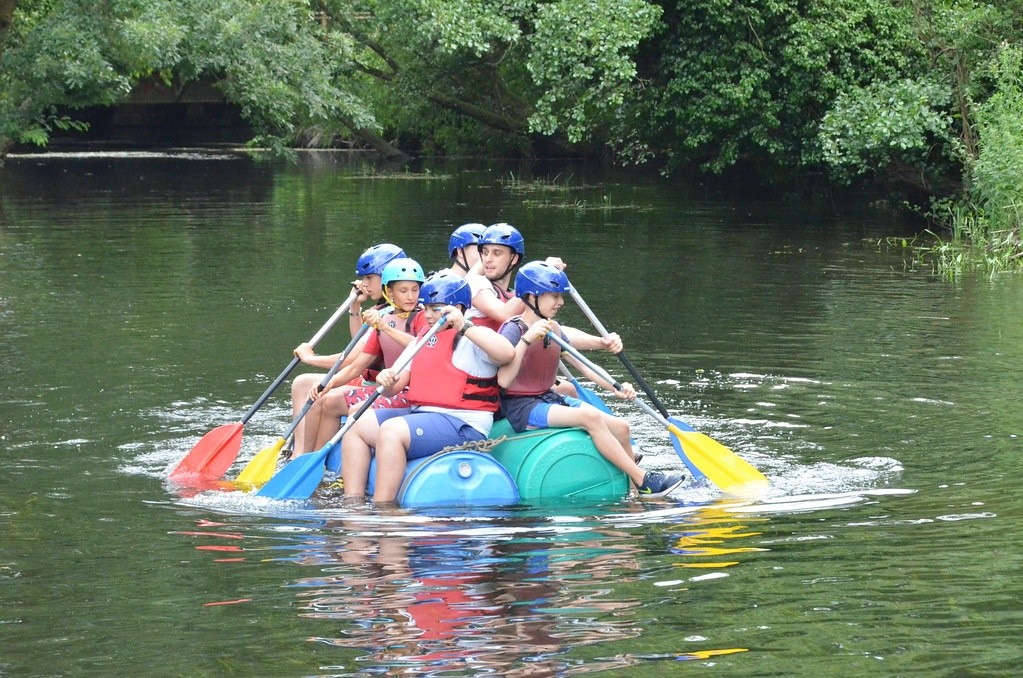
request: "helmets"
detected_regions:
[381,258,424,290]
[448,222,488,258]
[417,272,471,309]
[477,223,525,262]
[514,260,569,298]
[355,243,407,278]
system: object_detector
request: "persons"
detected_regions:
[302,258,425,454]
[444,223,488,279]
[341,269,516,504]
[495,261,686,497]
[288,243,406,462]
[463,222,644,465]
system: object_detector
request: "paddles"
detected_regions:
[168,279,364,480]
[255,307,448,500]
[557,357,636,446]
[567,278,719,483]
[234,319,377,486]
[538,328,771,497]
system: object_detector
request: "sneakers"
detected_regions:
[635,468,686,500]
[633,448,643,465]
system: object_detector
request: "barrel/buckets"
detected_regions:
[322,416,521,506]
[489,419,630,506]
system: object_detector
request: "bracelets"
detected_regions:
[521,335,531,346]
[349,307,362,316]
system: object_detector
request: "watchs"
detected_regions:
[461,320,472,335]
